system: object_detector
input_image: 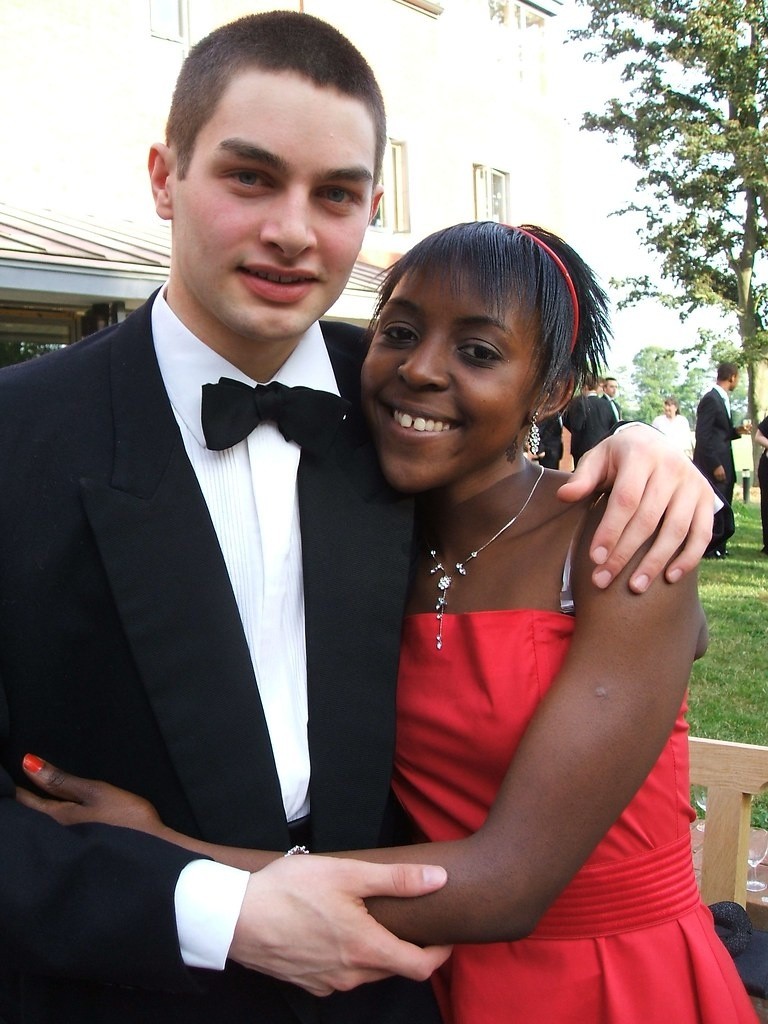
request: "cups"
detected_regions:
[743,419,751,430]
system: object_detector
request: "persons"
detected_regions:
[755,415,768,555]
[524,377,619,472]
[0,11,720,1024]
[653,399,692,459]
[693,362,753,558]
[17,222,759,1024]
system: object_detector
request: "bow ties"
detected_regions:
[201,377,353,464]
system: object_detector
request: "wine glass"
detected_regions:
[693,784,708,832]
[747,827,768,892]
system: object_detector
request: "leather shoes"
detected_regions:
[705,549,729,559]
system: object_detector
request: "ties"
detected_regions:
[612,400,621,420]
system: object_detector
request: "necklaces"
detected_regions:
[423,467,544,649]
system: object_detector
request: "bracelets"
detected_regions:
[285,846,309,856]
